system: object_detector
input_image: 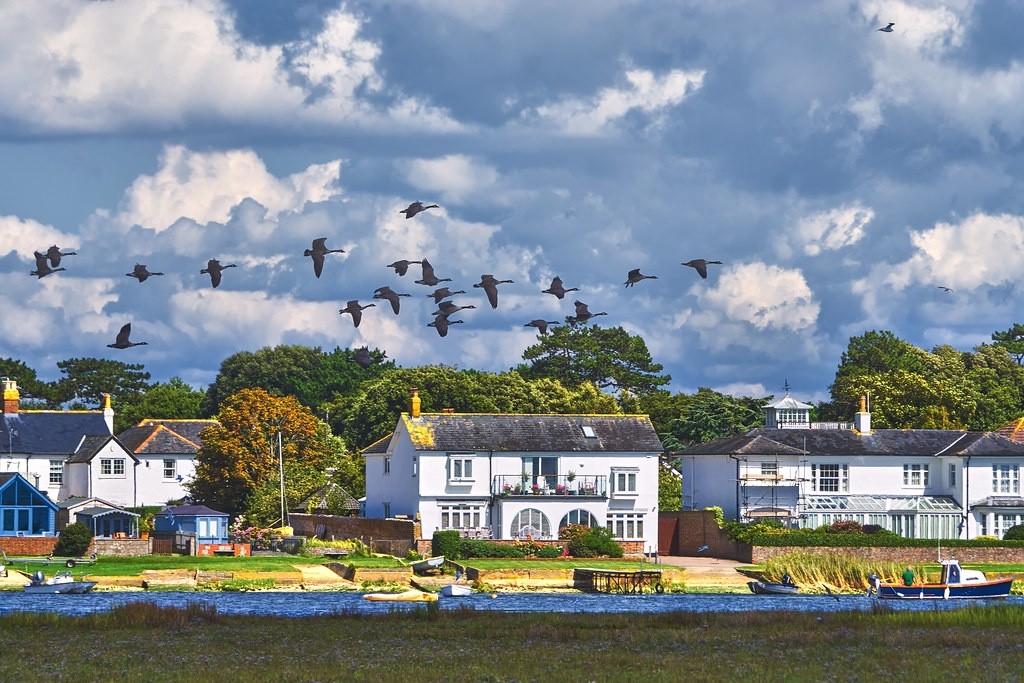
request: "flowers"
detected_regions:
[529,484,539,490]
[503,480,513,497]
[556,483,565,491]
[591,487,598,491]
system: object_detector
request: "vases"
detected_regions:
[568,490,576,495]
[533,488,539,495]
[555,490,561,495]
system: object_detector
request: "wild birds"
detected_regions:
[681,259,723,279]
[30,245,77,279]
[431,301,477,318]
[473,275,515,309]
[624,269,658,288]
[541,276,580,299]
[106,322,149,349]
[200,258,237,288]
[387,260,422,277]
[875,23,895,32]
[373,286,412,315]
[399,200,440,220]
[126,263,165,283]
[426,287,467,304]
[414,258,453,286]
[303,237,346,279]
[427,315,465,337]
[339,300,376,328]
[524,320,560,336]
[571,300,608,322]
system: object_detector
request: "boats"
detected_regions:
[441,585,472,597]
[408,555,447,576]
[747,580,802,595]
[868,559,1015,599]
[362,589,438,602]
[23,571,97,595]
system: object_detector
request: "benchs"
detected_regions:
[324,552,349,556]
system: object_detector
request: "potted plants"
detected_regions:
[521,471,532,482]
[520,485,528,495]
[567,470,576,483]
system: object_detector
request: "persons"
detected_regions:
[901,565,918,587]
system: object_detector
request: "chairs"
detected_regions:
[536,476,550,495]
